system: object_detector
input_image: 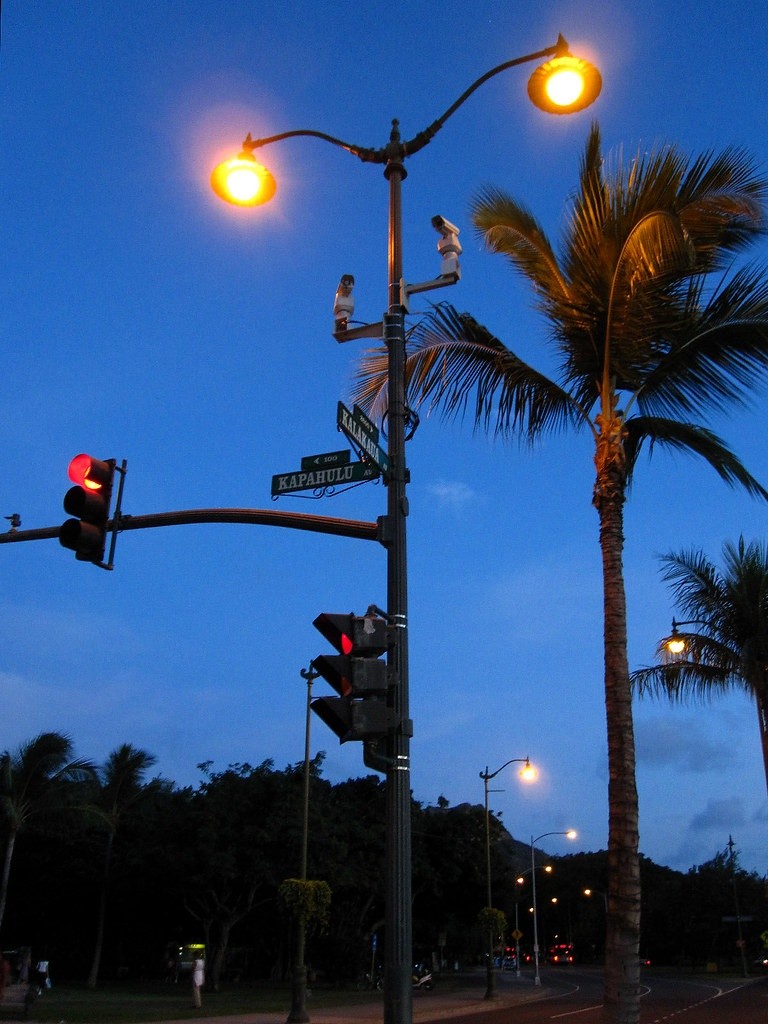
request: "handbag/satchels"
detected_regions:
[45,977,51,989]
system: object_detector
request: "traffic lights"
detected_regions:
[305,608,393,748]
[58,453,118,566]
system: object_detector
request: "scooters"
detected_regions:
[412,963,433,993]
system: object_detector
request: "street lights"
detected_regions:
[515,865,553,979]
[207,34,605,1023]
[478,756,538,952]
[527,830,577,986]
[666,615,768,792]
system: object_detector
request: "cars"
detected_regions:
[549,952,574,966]
[0,950,39,1017]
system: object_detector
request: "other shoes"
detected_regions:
[36,988,41,995]
[190,1005,200,1009]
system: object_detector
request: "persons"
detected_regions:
[162,950,205,1009]
[0,948,50,1002]
